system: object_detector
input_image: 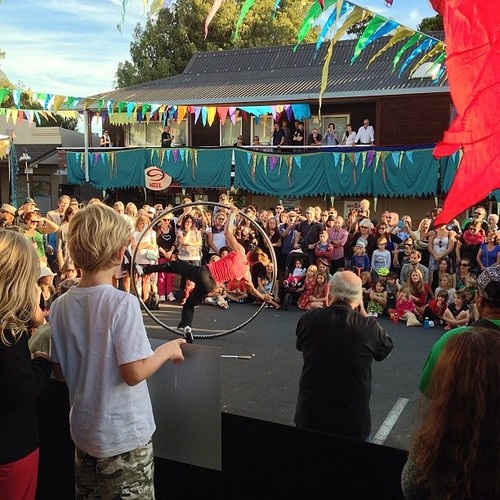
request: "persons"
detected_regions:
[353,119,374,146]
[292,122,304,146]
[307,128,322,146]
[295,270,394,500]
[339,124,357,145]
[0,191,500,330]
[401,326,500,500]
[253,136,261,146]
[234,136,245,146]
[282,122,291,146]
[161,126,173,148]
[273,124,285,146]
[324,123,337,145]
[418,265,500,397]
[100,130,110,147]
[0,230,50,500]
[48,203,187,500]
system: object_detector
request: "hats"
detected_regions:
[476,263,500,301]
[37,267,57,281]
[320,258,329,266]
[306,207,314,213]
[147,207,155,214]
[0,203,16,216]
[22,198,37,206]
[356,242,366,248]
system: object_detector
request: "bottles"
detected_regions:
[394,313,398,323]
[424,317,428,328]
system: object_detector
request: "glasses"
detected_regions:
[163,219,170,222]
[66,269,77,273]
[378,227,387,230]
[378,243,384,245]
[459,263,467,267]
[439,240,442,248]
[218,218,225,221]
[361,225,368,229]
[28,219,41,224]
[404,243,412,247]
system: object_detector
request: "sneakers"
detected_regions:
[168,293,176,301]
[159,295,165,301]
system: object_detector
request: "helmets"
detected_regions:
[378,268,389,275]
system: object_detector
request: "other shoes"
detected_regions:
[184,326,194,345]
[217,298,230,309]
[135,265,144,277]
[444,325,451,330]
